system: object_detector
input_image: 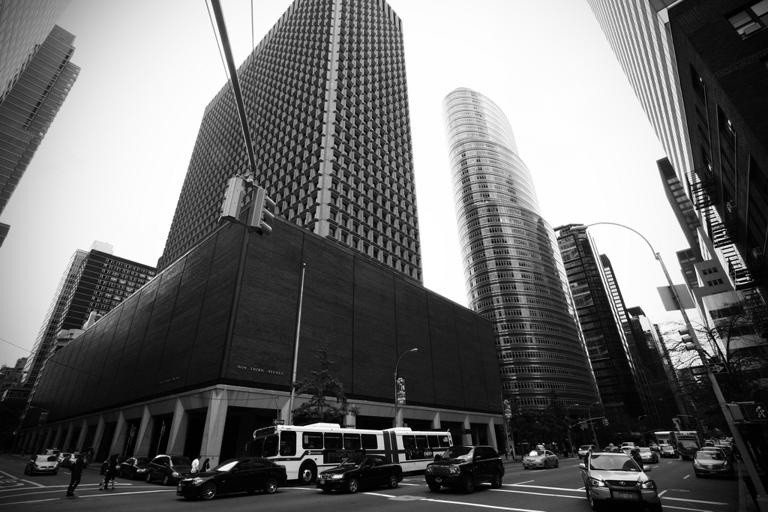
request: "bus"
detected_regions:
[241,421,454,489]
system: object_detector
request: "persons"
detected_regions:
[66,445,121,499]
[190,455,209,474]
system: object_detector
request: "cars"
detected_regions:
[691,437,737,481]
[175,457,290,498]
[42,448,87,469]
[575,448,663,511]
[573,440,680,464]
[316,453,405,494]
[521,448,561,470]
[24,454,60,476]
[98,453,195,488]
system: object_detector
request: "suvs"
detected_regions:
[422,444,506,492]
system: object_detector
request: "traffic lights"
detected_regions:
[603,418,610,426]
[724,402,744,424]
[746,402,767,422]
[679,326,698,352]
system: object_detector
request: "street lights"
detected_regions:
[565,402,579,460]
[567,219,765,505]
[588,399,601,451]
[638,414,648,447]
[390,345,423,429]
[255,195,280,241]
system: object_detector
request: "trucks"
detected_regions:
[668,430,700,463]
